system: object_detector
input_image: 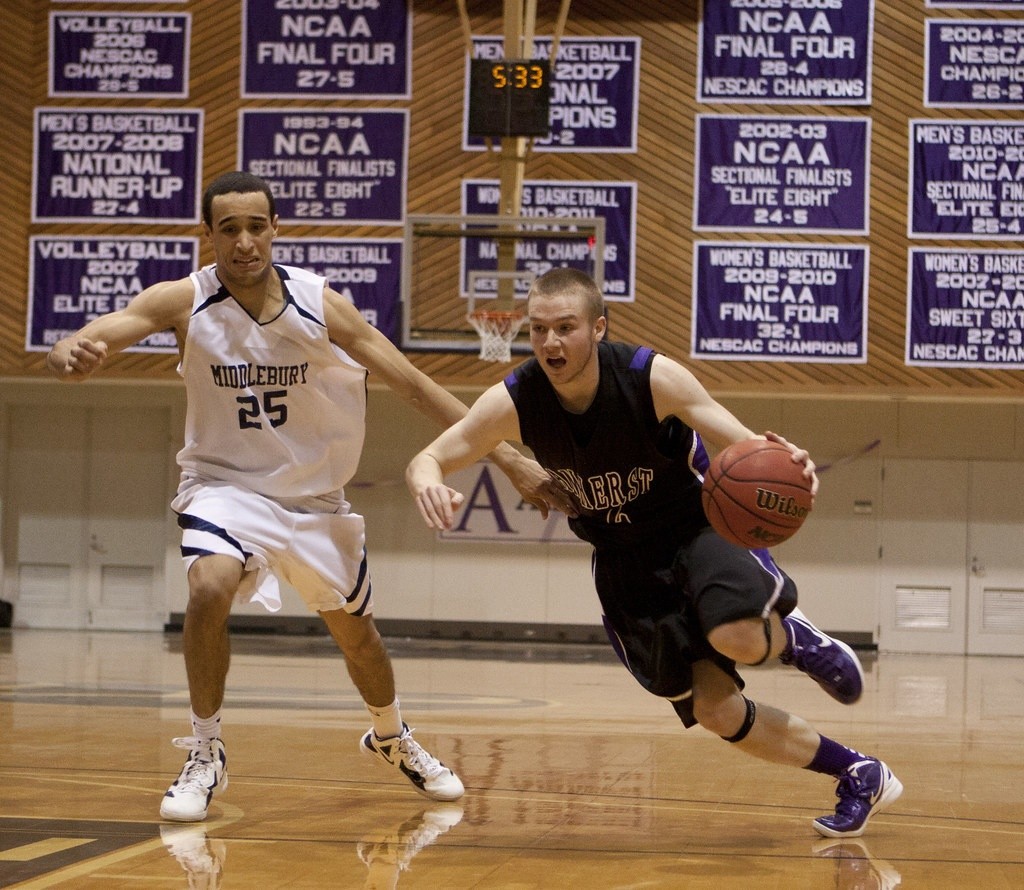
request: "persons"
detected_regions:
[46,171,581,823]
[812,838,901,890]
[160,804,464,890]
[405,266,905,838]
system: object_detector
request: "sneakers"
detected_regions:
[159,825,224,890]
[356,805,464,871]
[359,722,465,801]
[781,607,864,704]
[813,756,903,839]
[159,734,227,821]
[812,837,901,890]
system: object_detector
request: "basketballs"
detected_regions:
[702,440,812,550]
